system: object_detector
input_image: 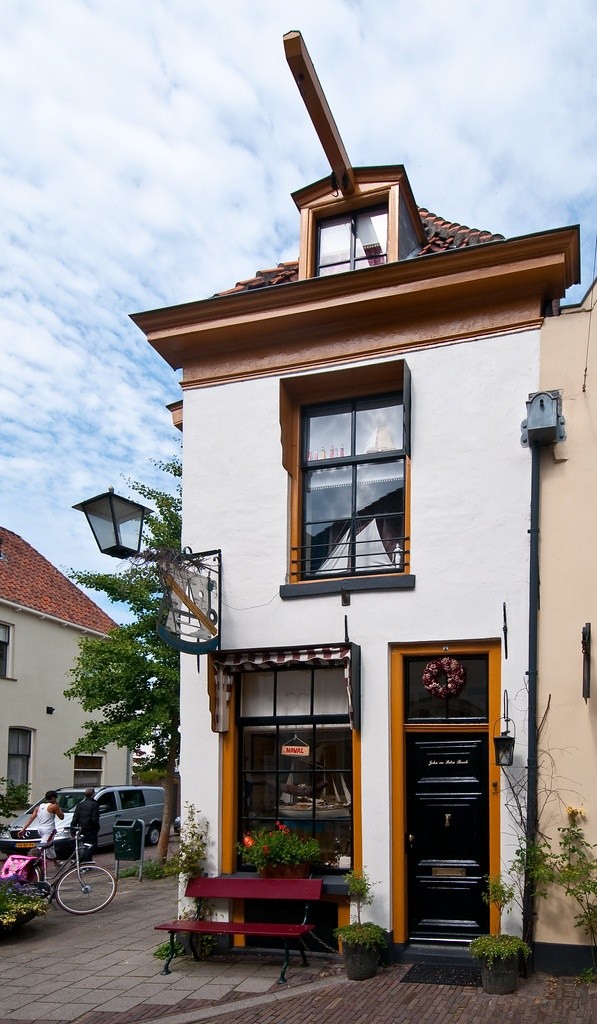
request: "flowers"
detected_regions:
[421,657,465,698]
[230,819,320,875]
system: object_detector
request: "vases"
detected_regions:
[257,860,312,879]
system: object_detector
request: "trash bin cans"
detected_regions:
[113,818,145,862]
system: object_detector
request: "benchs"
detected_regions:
[153,878,323,984]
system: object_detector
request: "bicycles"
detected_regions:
[0,823,118,915]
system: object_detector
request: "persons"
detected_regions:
[71,788,101,872]
[17,791,64,867]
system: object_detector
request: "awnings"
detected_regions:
[212,646,353,731]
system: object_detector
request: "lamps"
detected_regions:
[493,689,516,766]
[71,486,202,568]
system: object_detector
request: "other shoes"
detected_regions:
[83,870,87,873]
[55,862,64,866]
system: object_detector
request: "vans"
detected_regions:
[0,784,166,859]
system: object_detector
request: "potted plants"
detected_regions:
[469,877,532,996]
[0,868,53,936]
[331,865,391,981]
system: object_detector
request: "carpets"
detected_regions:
[400,963,484,987]
